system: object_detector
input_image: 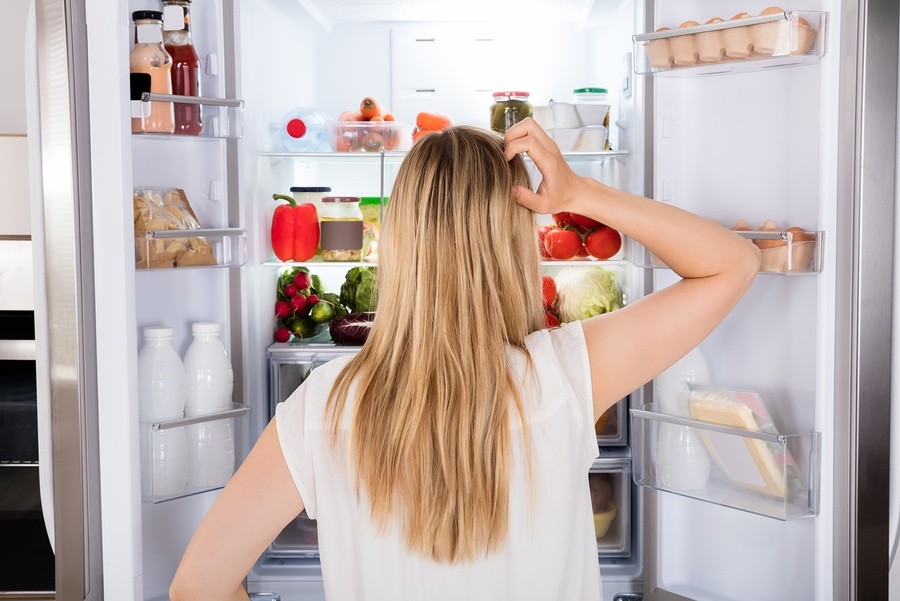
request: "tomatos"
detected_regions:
[536,211,622,261]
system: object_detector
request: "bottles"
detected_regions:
[284,111,330,153]
[137,324,189,498]
[130,9,175,135]
[181,322,236,488]
[161,0,203,135]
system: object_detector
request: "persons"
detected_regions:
[163,110,765,601]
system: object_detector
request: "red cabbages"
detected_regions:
[331,312,375,347]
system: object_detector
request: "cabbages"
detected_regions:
[554,265,625,323]
[339,267,380,313]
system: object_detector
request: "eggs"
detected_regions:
[733,218,812,248]
[651,5,809,39]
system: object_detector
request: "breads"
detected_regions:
[133,191,212,267]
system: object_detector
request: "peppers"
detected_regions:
[271,193,319,262]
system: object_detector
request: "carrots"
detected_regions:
[334,96,399,153]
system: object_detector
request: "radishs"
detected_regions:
[274,266,344,343]
